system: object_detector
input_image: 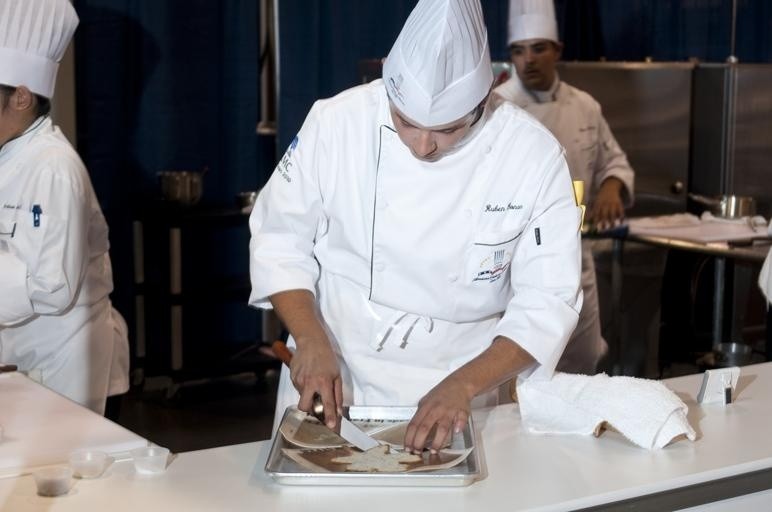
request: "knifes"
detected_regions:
[268,338,404,461]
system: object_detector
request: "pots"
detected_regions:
[689,192,758,217]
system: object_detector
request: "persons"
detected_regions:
[246,57,587,460]
[0,84,134,427]
[490,40,638,376]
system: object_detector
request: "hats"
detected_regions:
[0,0,80,99]
[383,0,493,127]
[506,0,559,47]
